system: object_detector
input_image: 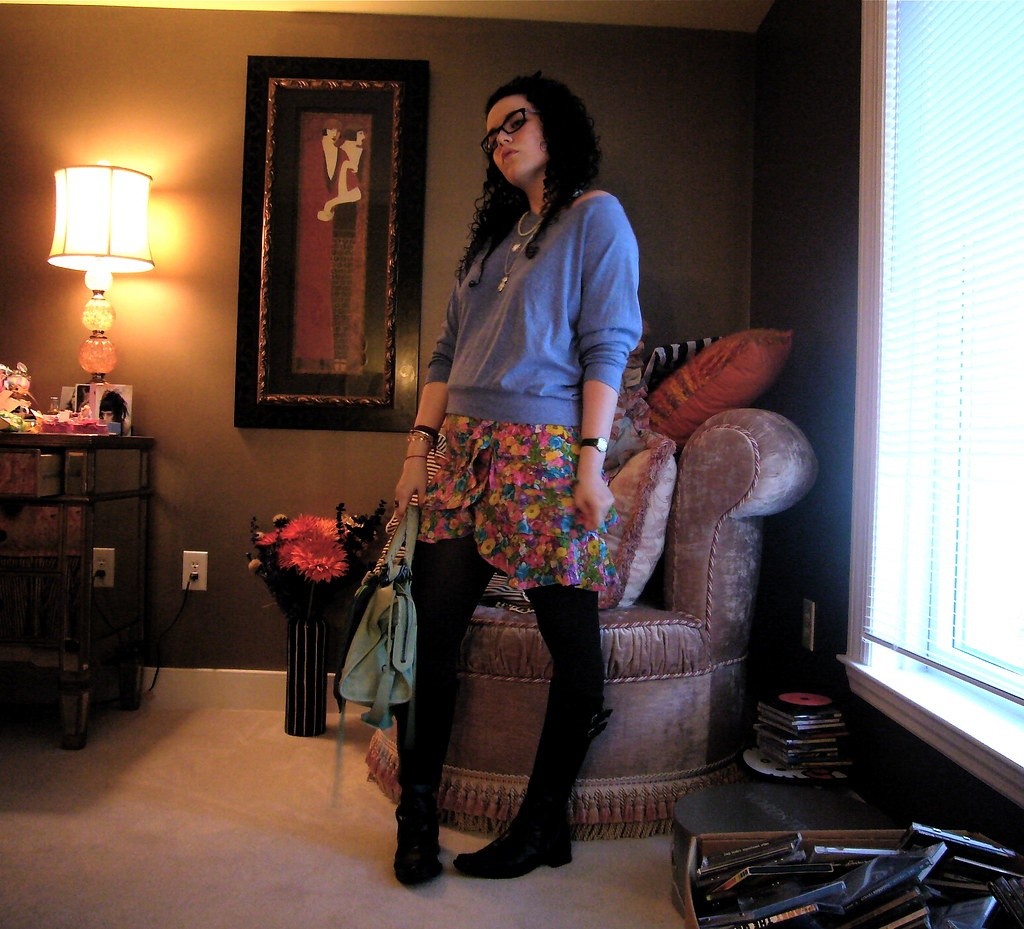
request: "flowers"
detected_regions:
[245,498,390,620]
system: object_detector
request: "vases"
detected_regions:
[285,616,328,737]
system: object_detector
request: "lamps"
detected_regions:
[45,164,154,381]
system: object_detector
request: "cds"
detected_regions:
[778,692,831,705]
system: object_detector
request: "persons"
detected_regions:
[394,76,642,886]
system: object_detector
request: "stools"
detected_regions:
[673,783,897,913]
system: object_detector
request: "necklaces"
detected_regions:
[497,210,544,293]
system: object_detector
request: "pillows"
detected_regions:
[464,325,792,614]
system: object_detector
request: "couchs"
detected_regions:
[361,408,821,841]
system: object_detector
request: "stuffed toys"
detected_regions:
[4,374,32,419]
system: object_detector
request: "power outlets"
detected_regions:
[90,546,115,588]
[181,550,209,592]
[800,598,814,650]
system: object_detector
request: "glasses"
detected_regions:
[479,108,539,157]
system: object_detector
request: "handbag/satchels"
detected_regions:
[337,506,418,727]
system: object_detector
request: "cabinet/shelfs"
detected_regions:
[0,434,158,750]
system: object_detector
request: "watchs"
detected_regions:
[580,438,608,451]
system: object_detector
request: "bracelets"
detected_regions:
[403,455,428,464]
[407,425,438,448]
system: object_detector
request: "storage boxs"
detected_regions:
[0,450,90,496]
[0,505,88,558]
[684,828,1024,929]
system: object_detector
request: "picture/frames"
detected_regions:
[233,55,433,428]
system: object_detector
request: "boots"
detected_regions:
[392,673,460,886]
[453,673,614,879]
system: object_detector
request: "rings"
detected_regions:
[394,500,400,508]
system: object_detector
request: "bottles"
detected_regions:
[48,396,60,414]
